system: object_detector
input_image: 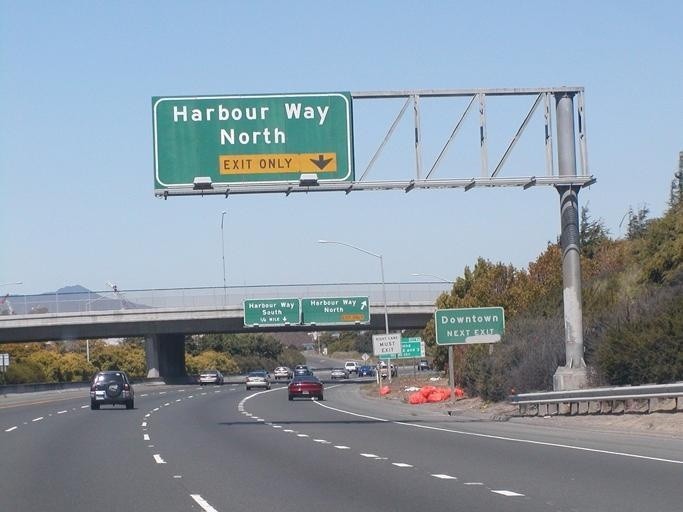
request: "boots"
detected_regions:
[90,370,134,409]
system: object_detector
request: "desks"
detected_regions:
[411,273,449,282]
[220,211,227,305]
[317,239,392,383]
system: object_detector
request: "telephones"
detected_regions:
[371,334,425,359]
[243,296,371,325]
[151,93,355,188]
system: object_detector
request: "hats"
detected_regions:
[274,361,394,380]
[288,375,324,401]
[199,370,224,386]
[246,372,271,390]
[418,359,433,371]
